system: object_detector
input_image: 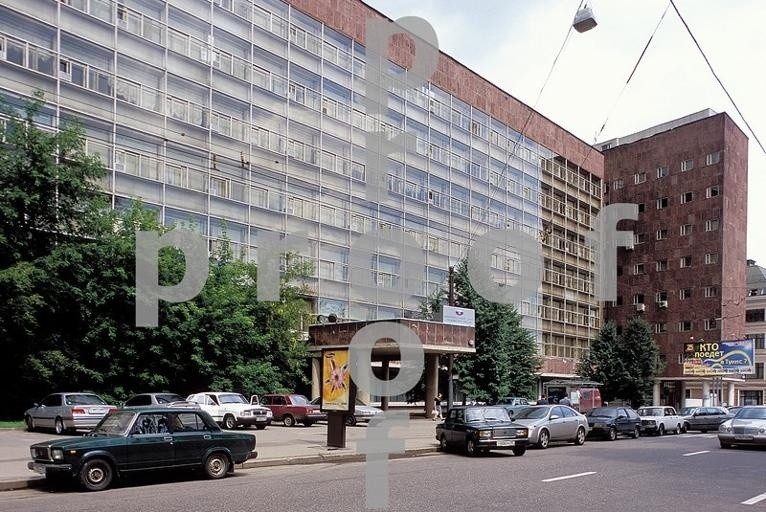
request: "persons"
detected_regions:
[433,393,446,421]
[536,395,549,405]
[559,392,573,407]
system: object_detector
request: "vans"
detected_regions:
[578,388,601,412]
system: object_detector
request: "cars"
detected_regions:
[727,406,743,415]
[436,406,529,456]
[23,392,118,434]
[186,391,273,431]
[494,396,531,419]
[677,406,735,433]
[259,392,327,427]
[310,396,385,426]
[116,392,204,428]
[717,405,765,449]
[512,404,590,448]
[27,404,261,492]
[636,406,682,435]
[585,406,643,440]
